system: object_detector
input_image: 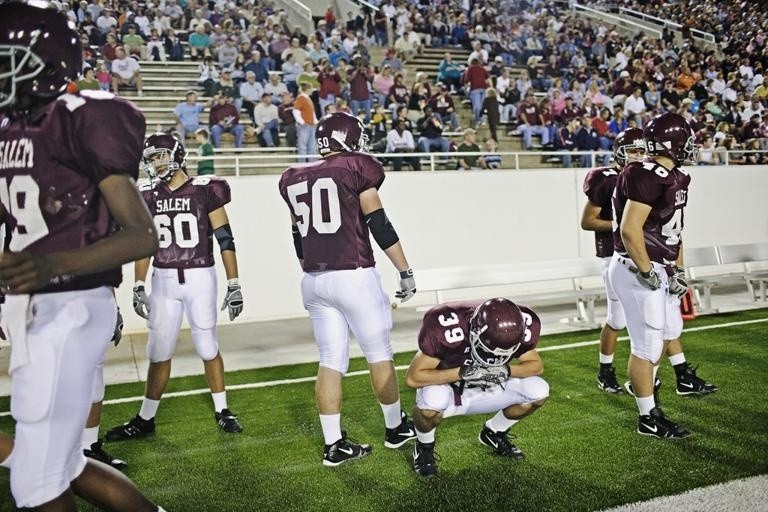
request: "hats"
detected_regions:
[85,2,630,102]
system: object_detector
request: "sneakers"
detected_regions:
[413,438,437,478]
[625,377,661,407]
[597,367,624,394]
[674,364,718,396]
[105,414,155,442]
[75,442,129,472]
[639,407,692,440]
[322,438,372,467]
[478,425,526,460]
[384,410,422,449]
[215,409,243,433]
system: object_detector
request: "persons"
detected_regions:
[579,127,723,398]
[80,302,127,474]
[0,431,14,469]
[278,113,419,468]
[54,2,767,174]
[606,113,695,441]
[405,298,551,478]
[0,0,173,512]
[105,130,247,441]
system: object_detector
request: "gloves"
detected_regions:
[458,364,511,388]
[665,266,688,299]
[395,268,416,303]
[640,266,661,290]
[221,281,243,321]
[106,305,124,347]
[132,285,150,322]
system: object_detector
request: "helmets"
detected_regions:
[315,111,364,156]
[643,113,696,168]
[613,126,650,167]
[469,298,523,368]
[0,2,81,113]
[141,133,186,183]
